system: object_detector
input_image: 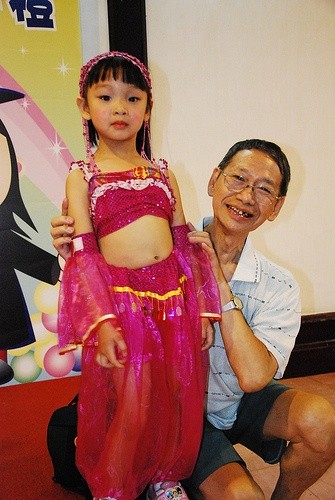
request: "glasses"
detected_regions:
[222,171,284,206]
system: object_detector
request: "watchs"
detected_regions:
[222,297,244,312]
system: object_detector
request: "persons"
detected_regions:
[57,51,222,500]
[47,139,335,500]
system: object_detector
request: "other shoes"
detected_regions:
[146,481,189,500]
